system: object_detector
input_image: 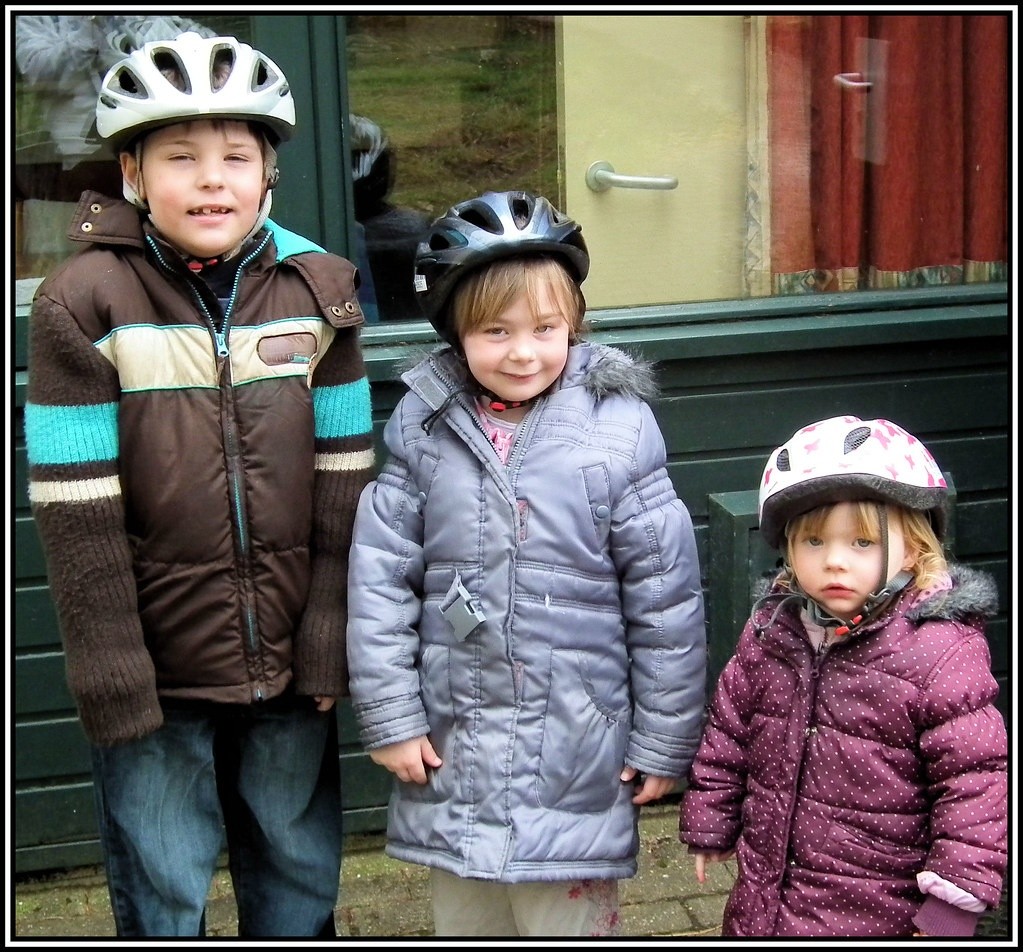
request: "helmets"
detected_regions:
[413,190,590,334]
[346,113,392,205]
[759,414,949,550]
[95,34,296,144]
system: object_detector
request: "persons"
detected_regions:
[29,35,376,935]
[347,192,705,937]
[679,416,1008,936]
[350,114,429,321]
